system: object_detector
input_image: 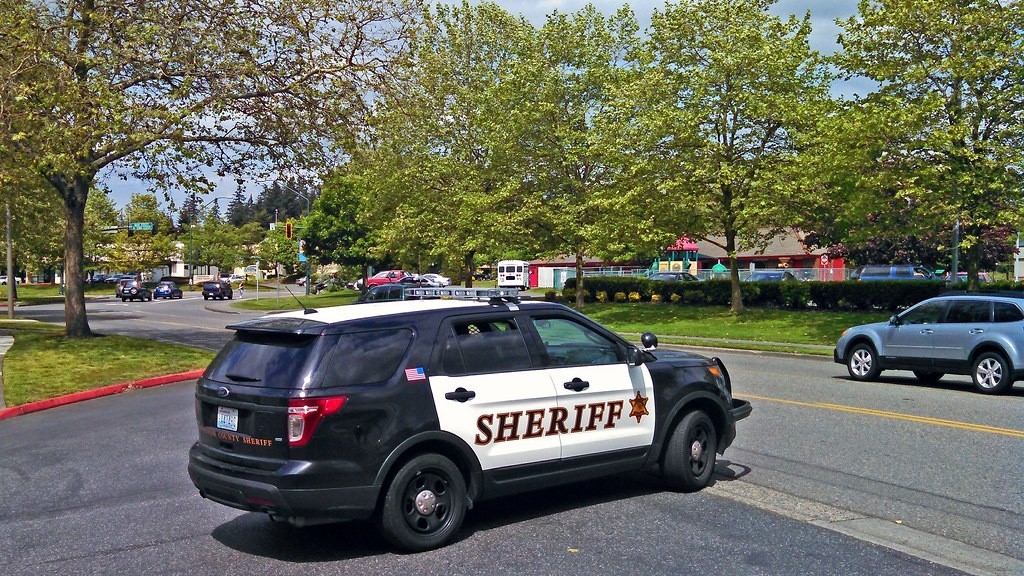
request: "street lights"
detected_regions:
[188,196,234,291]
[266,177,310,294]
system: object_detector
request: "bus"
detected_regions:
[497,259,531,292]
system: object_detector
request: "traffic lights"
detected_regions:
[285,222,292,239]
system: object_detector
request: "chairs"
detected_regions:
[958,313,972,323]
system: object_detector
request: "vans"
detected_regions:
[848,263,943,282]
[351,282,434,305]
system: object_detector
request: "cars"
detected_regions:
[396,276,443,288]
[153,281,184,299]
[744,270,797,282]
[295,276,311,287]
[647,271,699,283]
[0,276,22,286]
[313,269,420,295]
[423,273,452,287]
[85,273,139,298]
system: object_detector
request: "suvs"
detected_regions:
[202,281,234,299]
[833,291,1024,396]
[122,280,153,303]
[186,288,753,552]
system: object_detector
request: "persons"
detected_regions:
[238,281,244,298]
